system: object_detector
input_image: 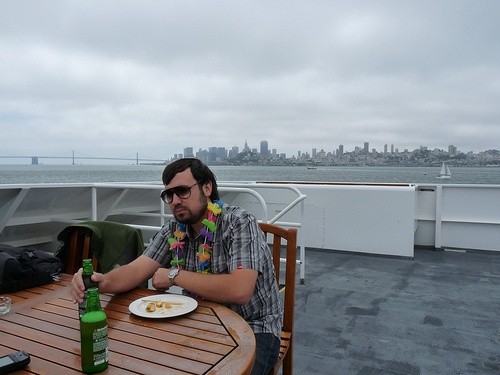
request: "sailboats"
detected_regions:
[436,161,451,179]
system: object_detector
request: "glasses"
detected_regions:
[160,179,203,204]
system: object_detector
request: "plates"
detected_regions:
[129,294,198,318]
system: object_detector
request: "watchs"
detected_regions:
[168,268,179,284]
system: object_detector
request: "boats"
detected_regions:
[307,167,317,169]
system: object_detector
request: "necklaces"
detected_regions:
[168,199,222,275]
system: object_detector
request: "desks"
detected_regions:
[0,273,256,375]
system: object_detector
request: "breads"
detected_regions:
[146,301,172,311]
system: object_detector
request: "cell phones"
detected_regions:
[0,351,30,373]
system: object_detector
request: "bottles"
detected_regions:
[78,259,97,321]
[80,288,109,374]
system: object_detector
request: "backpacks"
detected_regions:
[0,243,63,295]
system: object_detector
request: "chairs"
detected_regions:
[258,220,297,375]
[54,220,148,289]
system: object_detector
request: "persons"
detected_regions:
[71,158,283,375]
